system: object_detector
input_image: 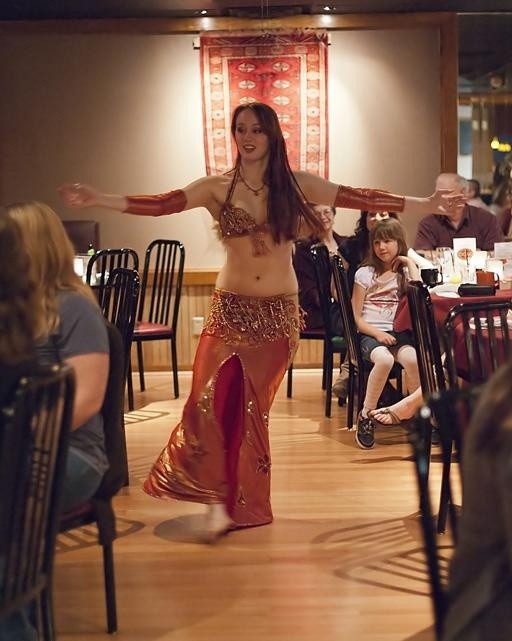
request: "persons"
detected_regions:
[52,104,467,540]
[451,361,510,639]
[466,178,492,212]
[295,173,507,449]
[0,198,111,522]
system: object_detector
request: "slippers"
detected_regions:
[367,406,416,428]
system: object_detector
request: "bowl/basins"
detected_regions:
[457,284,496,297]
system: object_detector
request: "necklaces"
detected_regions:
[236,162,270,198]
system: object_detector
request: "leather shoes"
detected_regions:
[333,369,349,398]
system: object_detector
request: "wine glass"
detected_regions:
[437,248,453,285]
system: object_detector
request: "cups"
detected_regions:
[466,250,487,281]
[493,242,512,282]
[485,259,504,282]
[420,267,443,288]
[452,237,476,283]
[476,272,499,291]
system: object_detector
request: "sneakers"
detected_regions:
[431,424,440,448]
[355,409,376,449]
